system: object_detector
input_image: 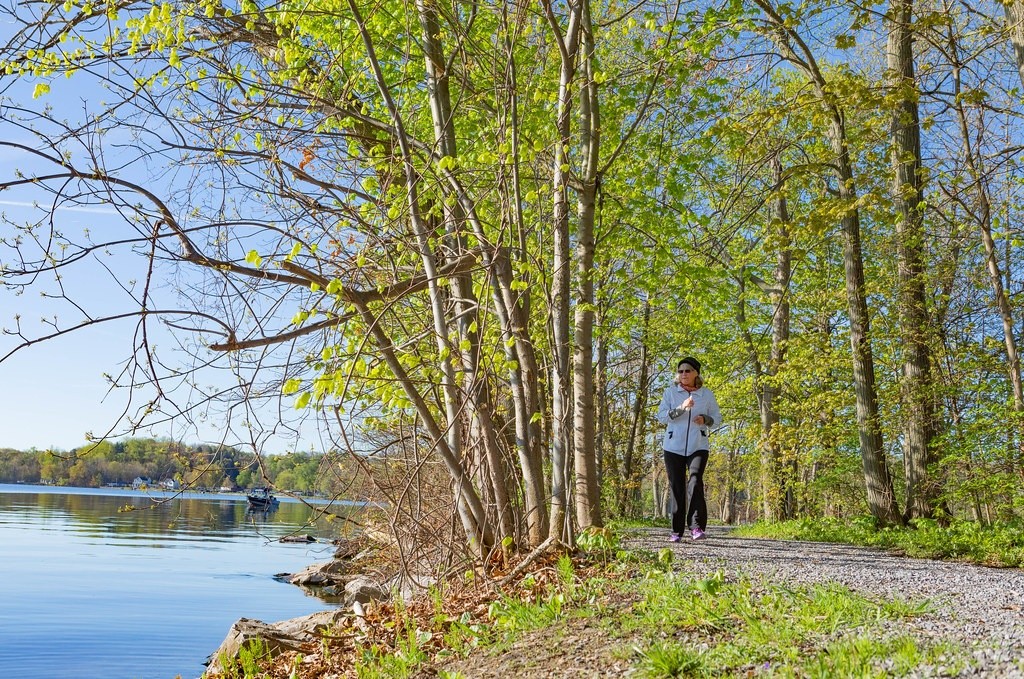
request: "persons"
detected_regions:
[657,357,721,543]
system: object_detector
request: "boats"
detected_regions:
[247,487,280,506]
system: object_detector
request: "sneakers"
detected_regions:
[668,533,682,543]
[689,526,707,540]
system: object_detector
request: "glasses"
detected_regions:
[678,369,690,373]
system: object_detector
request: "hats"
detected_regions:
[678,357,701,375]
